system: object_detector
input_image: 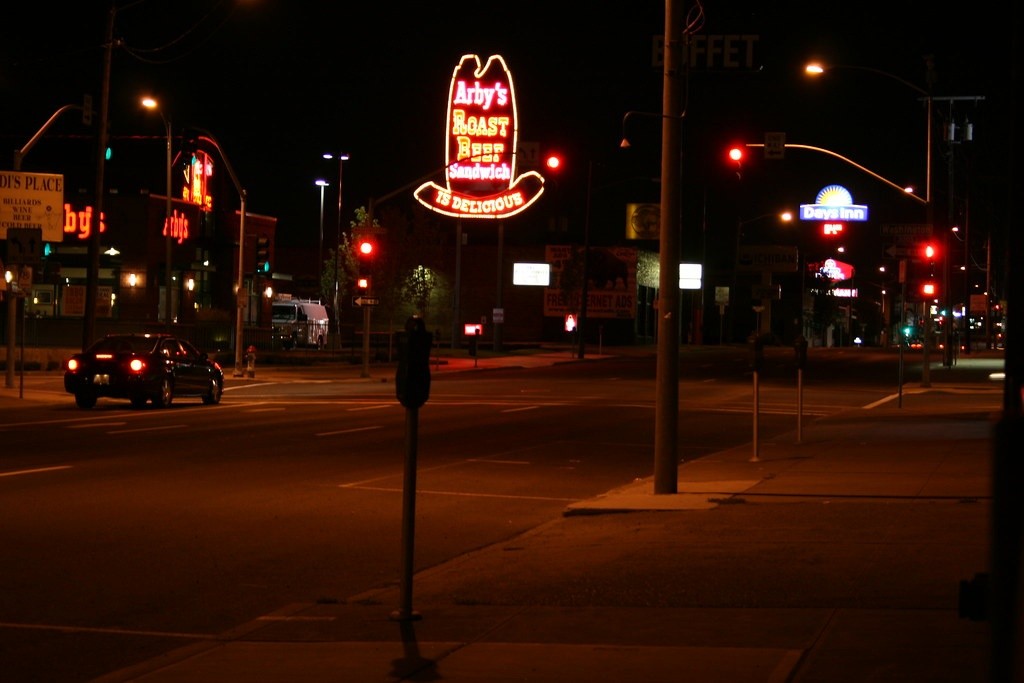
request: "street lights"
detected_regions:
[141,98,172,333]
[316,180,329,298]
[805,61,932,390]
[323,152,350,350]
[953,226,970,354]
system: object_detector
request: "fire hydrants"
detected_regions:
[245,345,257,378]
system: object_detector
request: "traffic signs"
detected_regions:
[352,296,380,307]
[882,224,932,259]
[764,133,785,158]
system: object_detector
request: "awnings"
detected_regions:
[272,272,304,290]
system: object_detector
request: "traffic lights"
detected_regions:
[359,241,376,258]
[545,154,562,172]
[728,147,744,162]
[922,284,936,295]
[357,277,370,289]
[922,244,938,260]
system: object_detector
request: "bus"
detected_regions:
[272,301,329,351]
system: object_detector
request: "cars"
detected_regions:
[64,334,225,411]
